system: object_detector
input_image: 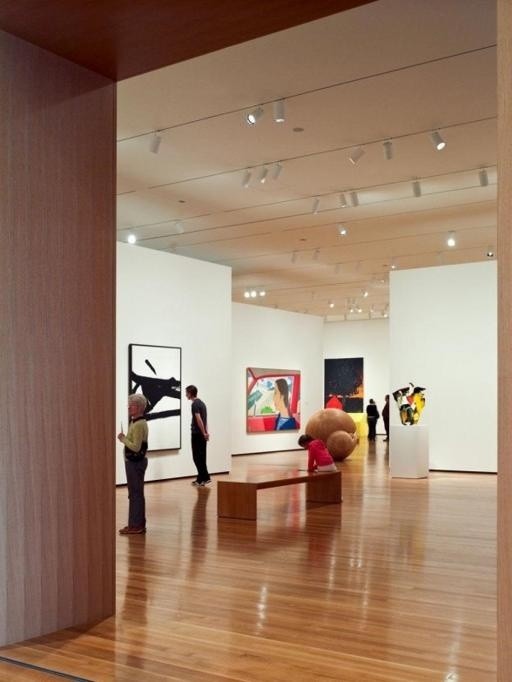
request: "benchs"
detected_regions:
[217,469,342,521]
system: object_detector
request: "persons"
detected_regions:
[118,394,148,535]
[187,385,212,485]
[367,399,379,440]
[382,395,390,441]
[299,434,337,472]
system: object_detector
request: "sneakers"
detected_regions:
[118,524,147,535]
[190,479,212,488]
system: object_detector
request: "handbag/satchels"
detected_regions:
[123,439,148,464]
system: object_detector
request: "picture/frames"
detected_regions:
[128,343,183,452]
